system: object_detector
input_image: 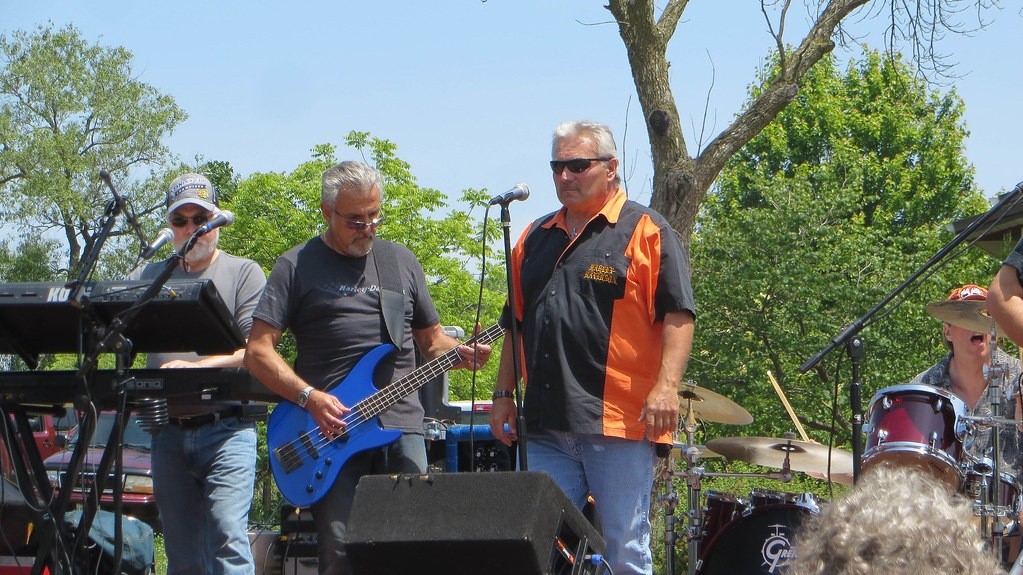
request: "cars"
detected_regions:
[0,406,162,537]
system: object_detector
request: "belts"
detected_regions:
[166,408,237,430]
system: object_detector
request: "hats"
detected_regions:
[164,174,220,217]
[946,282,989,301]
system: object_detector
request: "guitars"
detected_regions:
[266,320,509,511]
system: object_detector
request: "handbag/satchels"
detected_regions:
[39,509,155,575]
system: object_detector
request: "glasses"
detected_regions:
[550,157,613,177]
[333,204,385,231]
[167,211,214,228]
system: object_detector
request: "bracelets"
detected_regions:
[492,391,514,401]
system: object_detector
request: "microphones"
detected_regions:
[196,210,234,235]
[136,228,174,266]
[489,183,531,206]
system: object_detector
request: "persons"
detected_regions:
[489,121,696,575]
[788,465,1008,575]
[123,173,267,575]
[243,160,493,575]
[986,234,1023,348]
[909,285,1023,513]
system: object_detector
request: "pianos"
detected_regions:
[0,279,294,575]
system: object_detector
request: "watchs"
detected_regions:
[296,387,314,407]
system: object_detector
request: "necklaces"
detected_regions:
[569,227,578,239]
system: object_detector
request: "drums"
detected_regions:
[695,505,821,575]
[697,489,757,561]
[786,493,819,504]
[750,487,788,510]
[861,383,973,494]
[960,464,1021,515]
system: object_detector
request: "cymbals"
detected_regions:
[672,440,721,458]
[707,436,853,474]
[925,300,1010,339]
[678,381,754,426]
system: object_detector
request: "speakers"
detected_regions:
[344,471,611,575]
